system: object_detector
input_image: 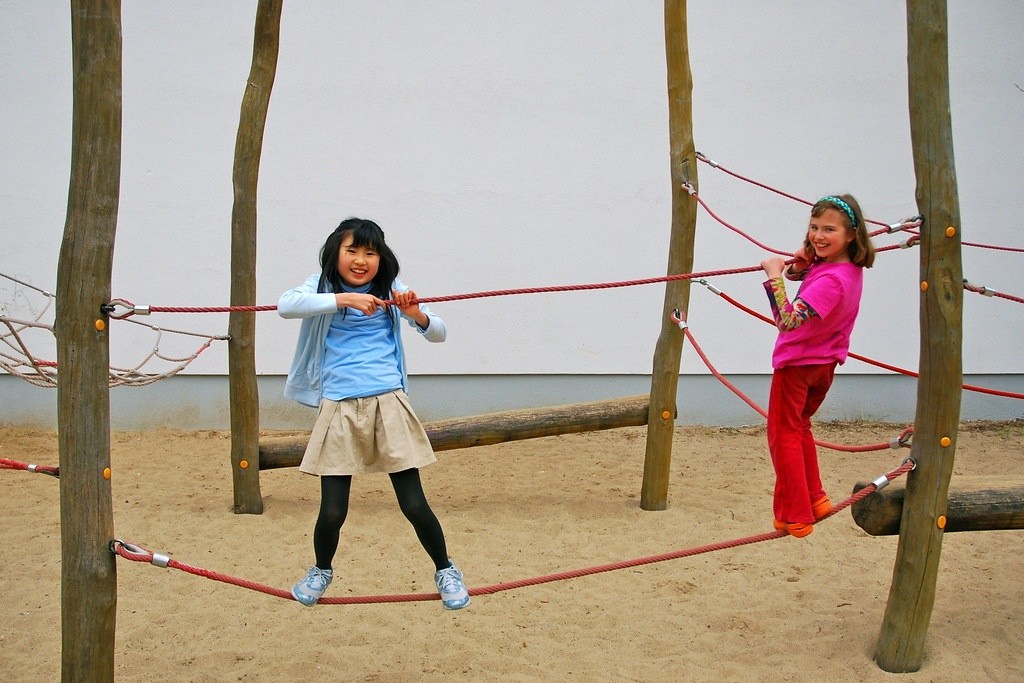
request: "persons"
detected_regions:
[762,193,876,538]
[277,217,471,611]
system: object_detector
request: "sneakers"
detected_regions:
[434,559,470,610]
[290,564,333,607]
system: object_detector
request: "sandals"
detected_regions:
[774,518,814,538]
[811,494,834,519]
